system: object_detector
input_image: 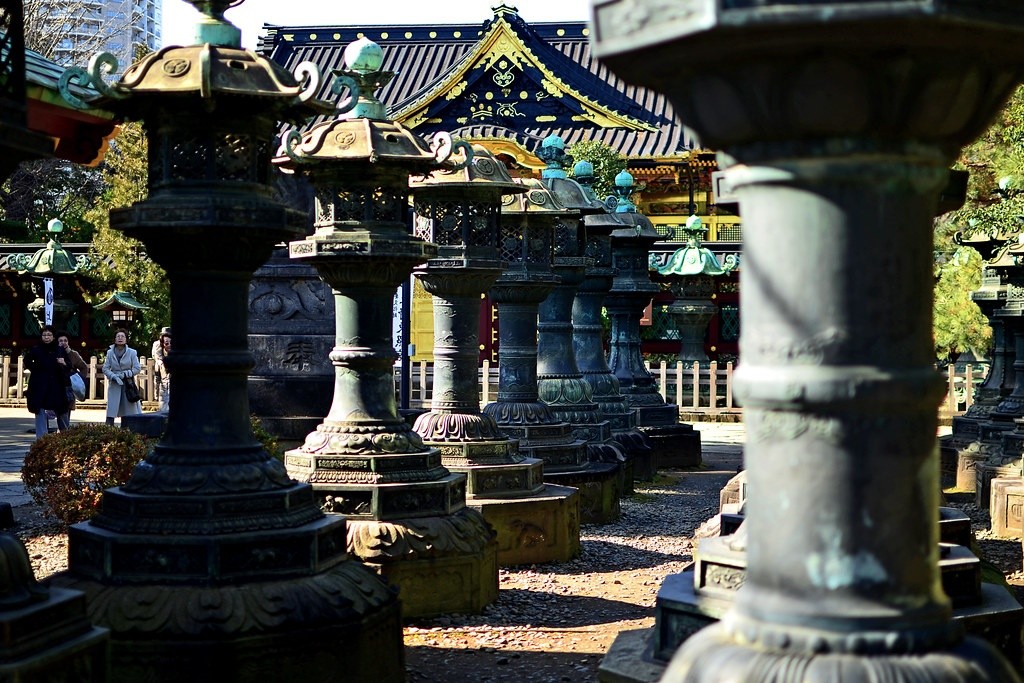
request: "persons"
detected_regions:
[151,326,170,411]
[23,325,87,440]
[102,329,142,426]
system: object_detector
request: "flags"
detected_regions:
[43,278,53,325]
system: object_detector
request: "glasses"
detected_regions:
[41,333,53,337]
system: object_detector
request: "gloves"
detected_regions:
[112,375,120,382]
[119,372,125,379]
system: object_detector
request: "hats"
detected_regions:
[160,326,172,335]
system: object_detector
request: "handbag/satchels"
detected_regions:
[123,368,139,403]
[70,372,86,402]
